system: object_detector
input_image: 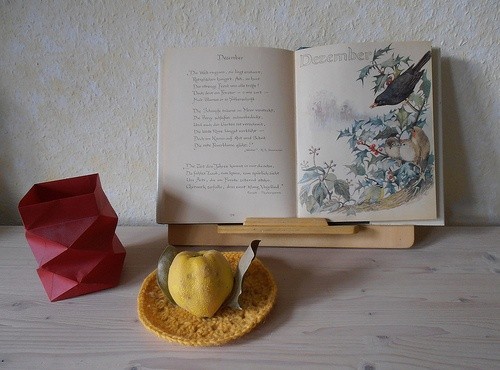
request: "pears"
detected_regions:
[167,249,234,319]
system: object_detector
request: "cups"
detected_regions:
[14,172,127,302]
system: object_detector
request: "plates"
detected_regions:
[136,251,278,346]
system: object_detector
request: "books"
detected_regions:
[154,39,446,227]
